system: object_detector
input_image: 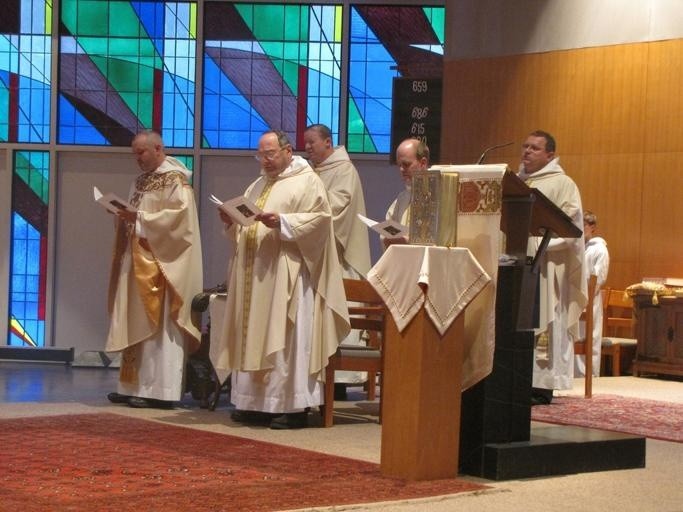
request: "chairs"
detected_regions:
[317,276,389,429]
[571,275,640,402]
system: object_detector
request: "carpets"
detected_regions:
[531,391,683,442]
[0,405,490,512]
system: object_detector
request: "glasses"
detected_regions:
[254,144,289,162]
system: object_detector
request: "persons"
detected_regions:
[108,131,203,409]
[221,129,351,424]
[515,130,610,404]
[302,125,371,401]
[381,138,429,246]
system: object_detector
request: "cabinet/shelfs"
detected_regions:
[627,292,682,381]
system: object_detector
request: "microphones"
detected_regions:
[477,140,515,165]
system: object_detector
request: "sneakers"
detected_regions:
[108,389,177,409]
[231,404,308,429]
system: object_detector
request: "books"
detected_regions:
[94,186,138,219]
[357,214,408,239]
[409,170,460,246]
[209,195,263,226]
